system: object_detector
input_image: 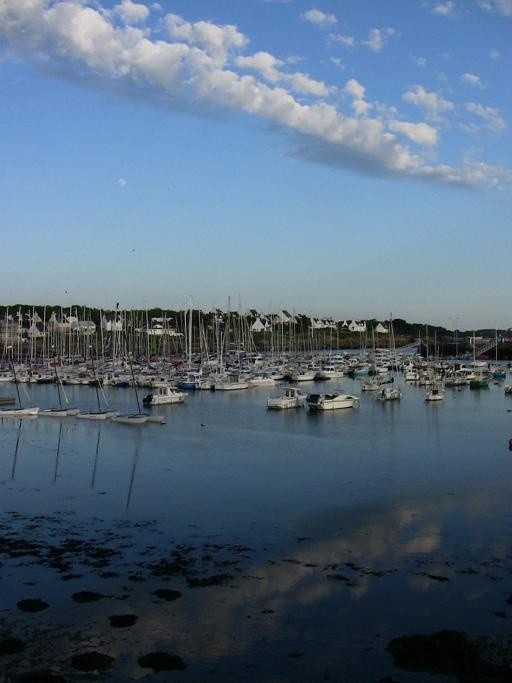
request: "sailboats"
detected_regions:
[2,343,164,424]
[336,296,512,391]
[1,300,344,392]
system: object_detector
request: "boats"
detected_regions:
[424,386,447,400]
[374,386,402,401]
[266,387,307,410]
[144,380,189,405]
[306,391,360,411]
